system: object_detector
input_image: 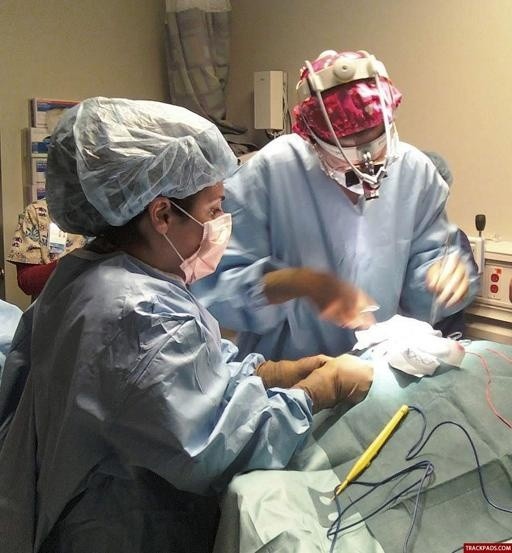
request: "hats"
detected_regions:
[290,51,402,142]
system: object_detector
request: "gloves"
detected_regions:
[256,353,373,414]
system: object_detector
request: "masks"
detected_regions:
[164,199,232,285]
[319,160,386,194]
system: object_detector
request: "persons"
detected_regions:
[6,199,88,303]
[0,93,379,551]
[184,45,487,362]
